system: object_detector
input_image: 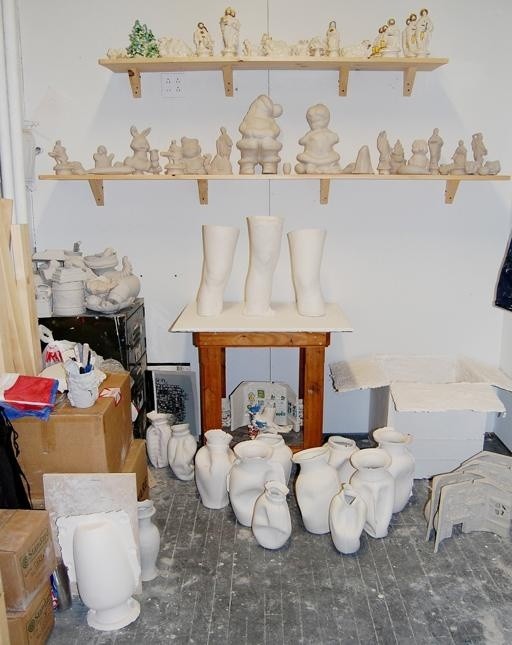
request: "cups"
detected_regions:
[64,358,99,408]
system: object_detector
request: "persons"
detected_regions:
[51,139,69,166]
[193,5,433,58]
[374,127,490,176]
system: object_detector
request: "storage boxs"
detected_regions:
[327,352,511,482]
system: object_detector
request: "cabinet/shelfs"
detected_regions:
[35,53,512,207]
[37,299,149,443]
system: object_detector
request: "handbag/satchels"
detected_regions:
[0,406,33,509]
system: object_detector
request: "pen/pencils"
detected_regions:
[80,366,86,374]
